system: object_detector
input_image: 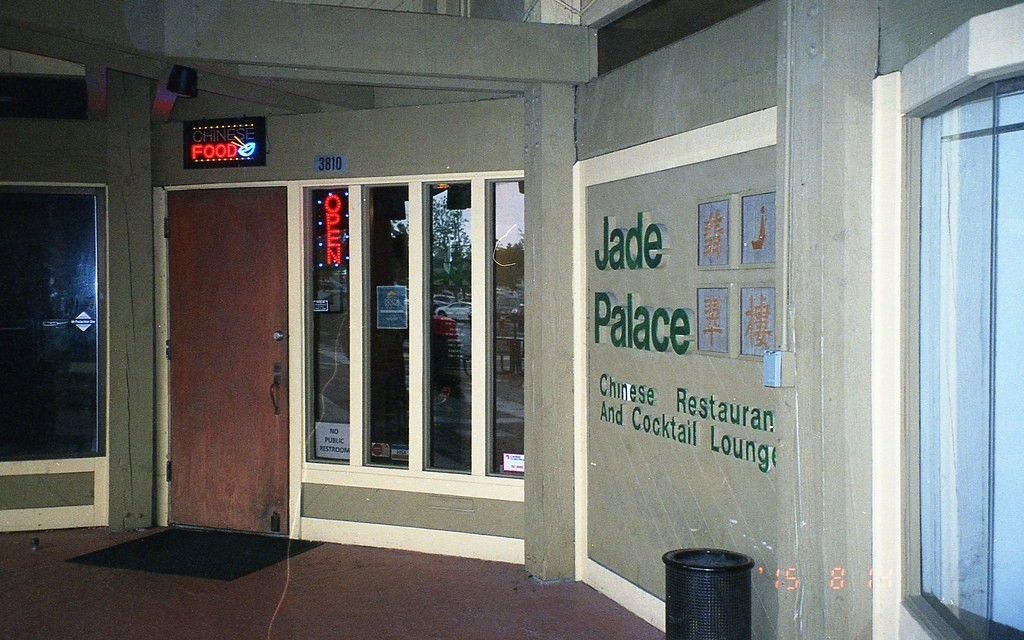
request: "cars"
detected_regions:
[433,300,449,308]
[434,301,471,321]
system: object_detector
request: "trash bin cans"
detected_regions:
[662,547,756,640]
